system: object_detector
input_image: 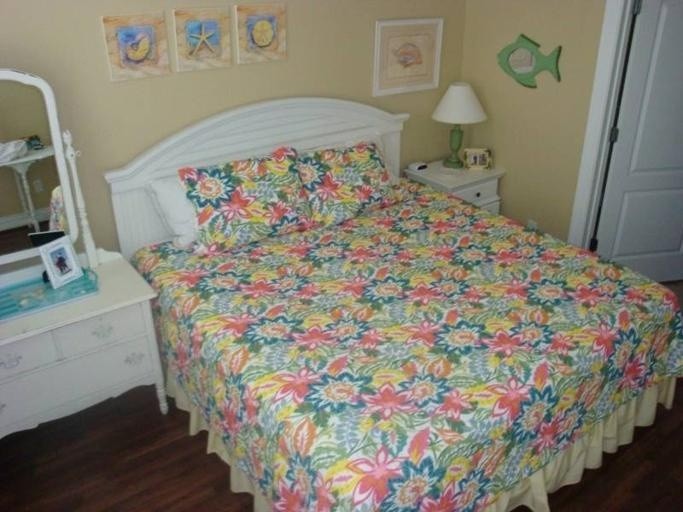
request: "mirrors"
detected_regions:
[0,69,103,288]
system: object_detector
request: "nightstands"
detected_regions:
[0,248,169,439]
[404,158,505,214]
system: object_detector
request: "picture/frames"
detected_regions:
[372,19,444,97]
[28,231,84,289]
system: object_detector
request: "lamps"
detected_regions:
[431,83,487,169]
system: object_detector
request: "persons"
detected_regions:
[54,252,70,274]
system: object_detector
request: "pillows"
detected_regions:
[147,135,407,253]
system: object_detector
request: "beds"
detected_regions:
[103,97,683,512]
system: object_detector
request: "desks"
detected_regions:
[0,142,55,234]
